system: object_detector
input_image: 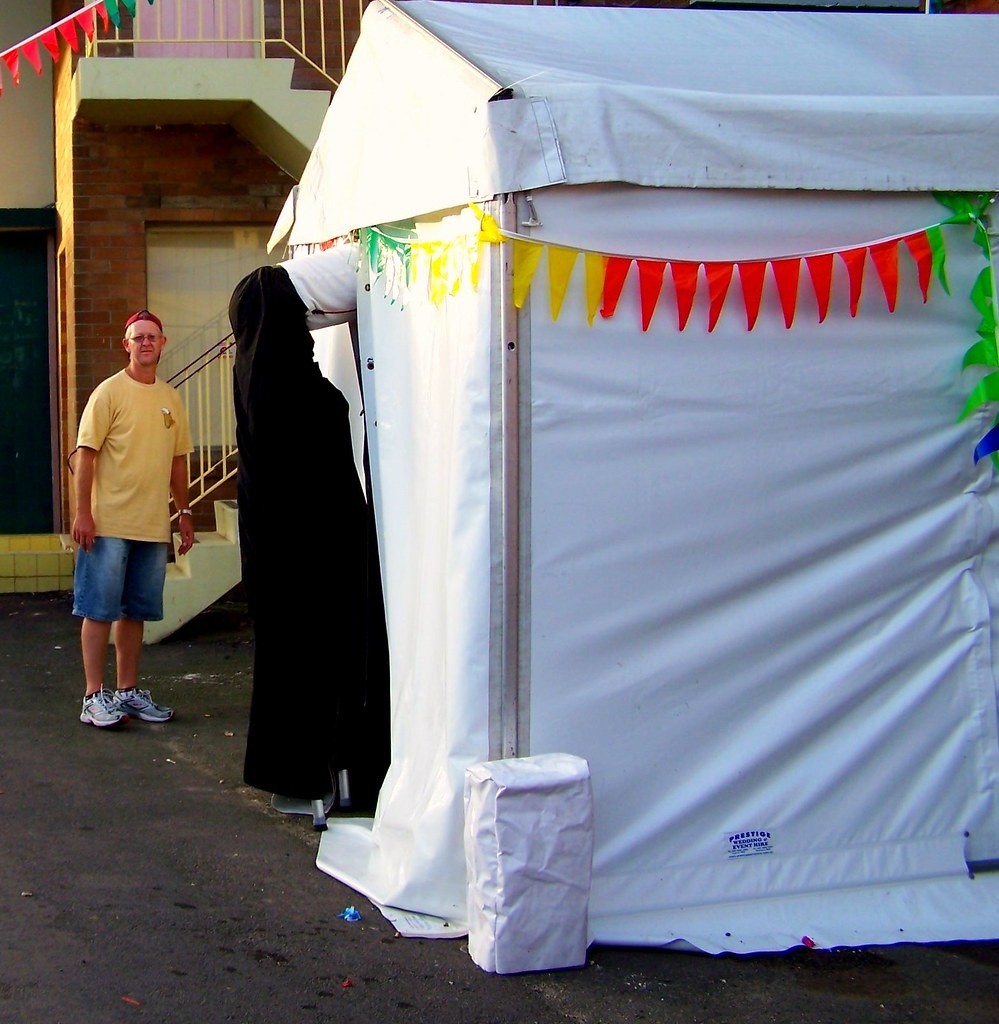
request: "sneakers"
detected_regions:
[113,688,176,722]
[79,683,129,728]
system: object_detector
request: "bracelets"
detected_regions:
[177,508,193,515]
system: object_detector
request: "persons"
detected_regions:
[71,309,196,728]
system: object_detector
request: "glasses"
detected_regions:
[129,332,163,341]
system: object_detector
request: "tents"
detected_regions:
[233,1,999,971]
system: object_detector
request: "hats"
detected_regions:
[124,309,162,332]
